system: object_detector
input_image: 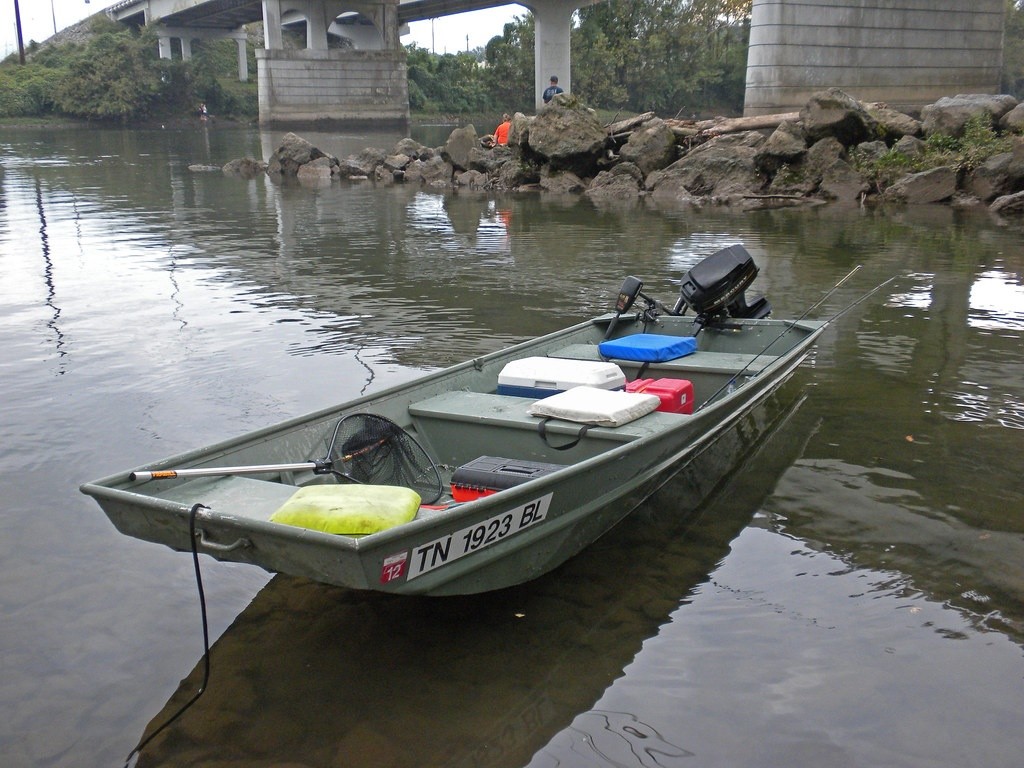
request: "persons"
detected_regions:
[199,103,207,116]
[543,76,563,104]
[498,209,512,234]
[492,113,512,148]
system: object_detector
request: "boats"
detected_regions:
[77,240,830,601]
[124,370,825,767]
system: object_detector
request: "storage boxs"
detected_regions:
[498,355,626,400]
[623,377,695,415]
[449,455,572,501]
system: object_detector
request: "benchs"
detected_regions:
[409,390,691,467]
[547,344,783,410]
